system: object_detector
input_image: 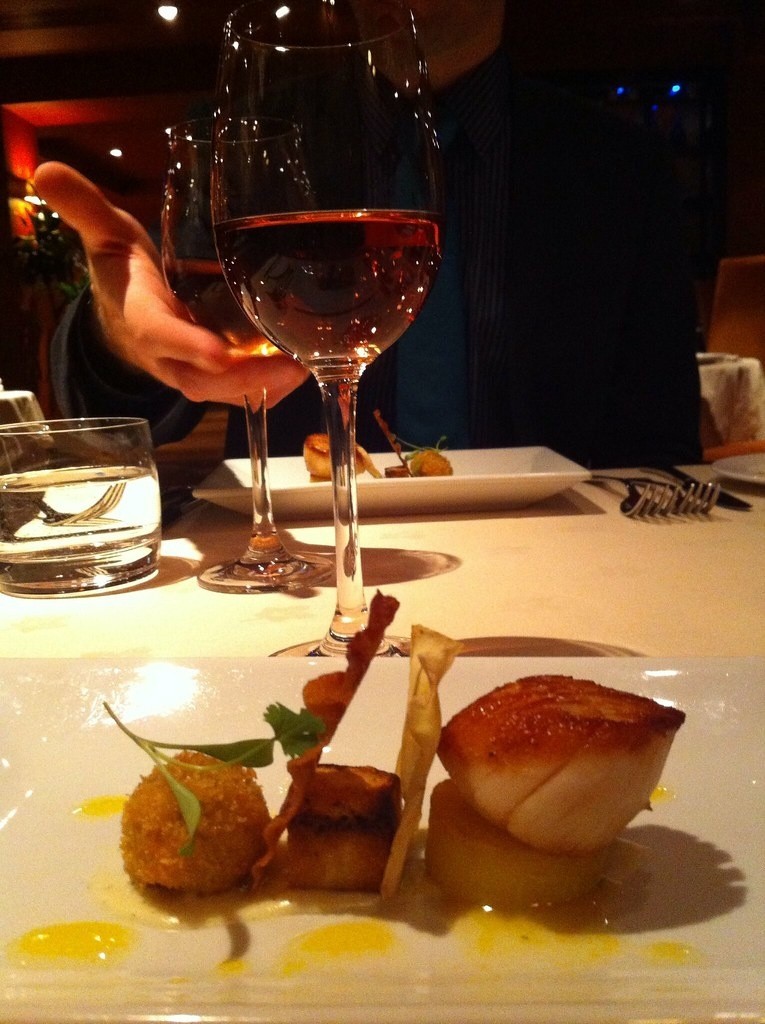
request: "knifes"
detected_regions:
[638,460,754,512]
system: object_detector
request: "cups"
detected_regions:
[0,418,164,600]
[0,390,54,513]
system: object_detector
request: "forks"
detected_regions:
[580,474,721,520]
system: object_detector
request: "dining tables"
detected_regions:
[0,467,765,659]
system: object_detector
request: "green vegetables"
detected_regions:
[391,434,449,476]
[103,700,327,855]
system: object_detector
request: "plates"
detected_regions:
[192,443,593,521]
[1,653,765,1024]
[711,451,765,487]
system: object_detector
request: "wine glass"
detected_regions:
[208,1,446,656]
[160,115,336,593]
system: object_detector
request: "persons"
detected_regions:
[17,0,727,471]
